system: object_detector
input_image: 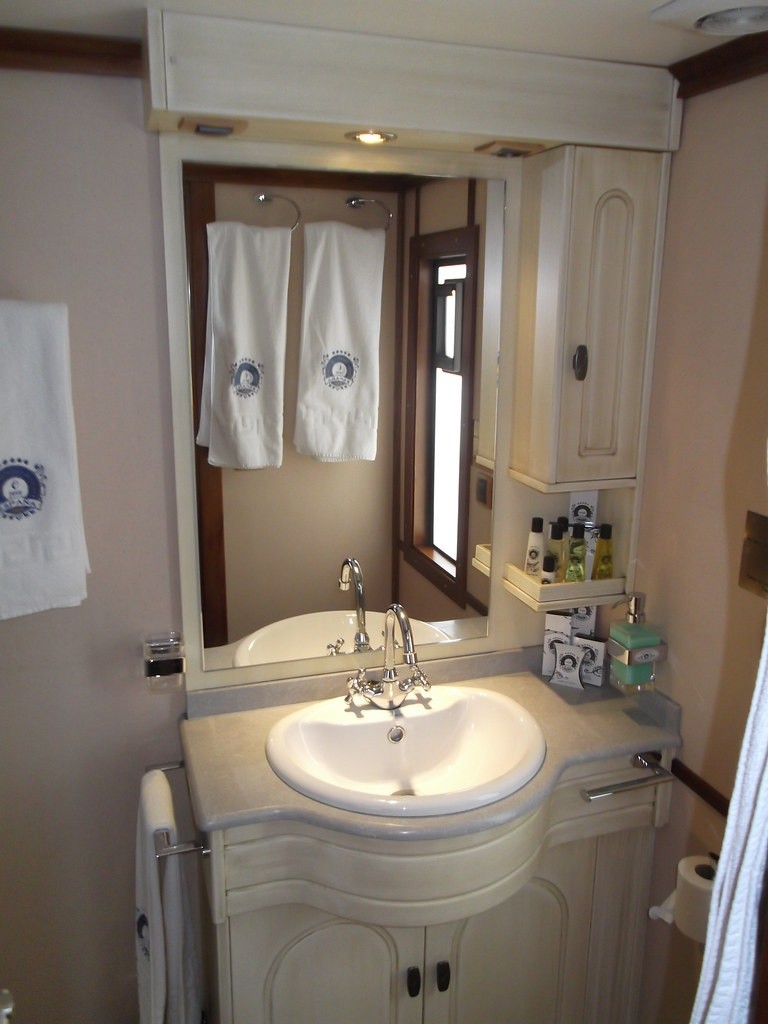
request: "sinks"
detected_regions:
[236,610,450,672]
[265,685,548,813]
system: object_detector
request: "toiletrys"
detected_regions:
[524,490,665,694]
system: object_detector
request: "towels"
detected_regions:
[296,222,387,466]
[2,302,90,623]
[133,767,189,1021]
[197,222,296,473]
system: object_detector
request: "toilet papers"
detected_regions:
[649,853,720,942]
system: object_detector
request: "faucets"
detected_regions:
[344,604,434,710]
[327,557,400,653]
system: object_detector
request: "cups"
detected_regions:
[145,632,184,694]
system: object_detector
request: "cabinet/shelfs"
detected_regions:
[211,799,654,1024]
[508,142,675,494]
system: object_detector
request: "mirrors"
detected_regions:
[181,160,506,672]
